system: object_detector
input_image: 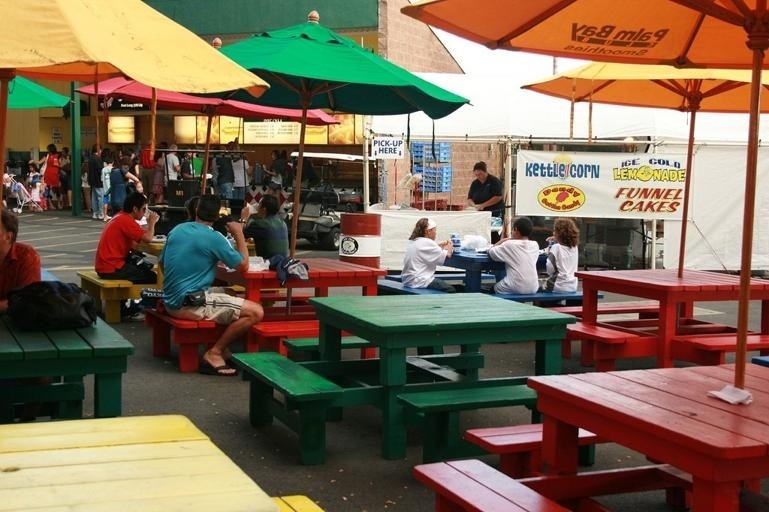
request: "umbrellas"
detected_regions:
[400,0,768,390]
[521,61,769,327]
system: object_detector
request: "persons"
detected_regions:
[0,204,41,311]
[159,194,264,374]
[400,217,456,293]
[467,161,505,244]
[152,143,312,216]
[29,145,71,211]
[487,217,540,295]
[94,193,159,313]
[242,195,289,259]
[533,218,580,306]
[79,145,143,223]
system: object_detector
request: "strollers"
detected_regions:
[10,178,43,214]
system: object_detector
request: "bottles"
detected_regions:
[224,232,234,248]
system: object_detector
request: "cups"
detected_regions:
[450,233,461,240]
[223,199,230,210]
[144,209,160,223]
[548,240,555,248]
[452,237,461,254]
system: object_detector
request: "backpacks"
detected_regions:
[5,280,99,333]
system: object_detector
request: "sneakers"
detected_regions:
[122,312,146,322]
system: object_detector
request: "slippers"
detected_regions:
[200,361,237,377]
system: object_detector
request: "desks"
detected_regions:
[574,269,769,369]
[307,288,579,461]
[367,206,492,279]
[1,306,136,423]
[527,363,769,512]
[0,412,281,512]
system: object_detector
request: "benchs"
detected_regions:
[281,335,487,388]
[232,351,346,465]
[562,322,640,373]
[396,381,538,467]
[466,421,615,479]
[412,459,573,512]
[548,300,661,321]
[252,319,353,357]
[269,492,323,512]
[682,335,769,365]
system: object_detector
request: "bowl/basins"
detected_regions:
[344,188,353,193]
[334,188,342,193]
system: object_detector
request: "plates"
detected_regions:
[389,205,401,210]
[151,239,166,243]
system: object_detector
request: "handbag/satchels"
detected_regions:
[120,169,136,195]
[60,170,67,181]
